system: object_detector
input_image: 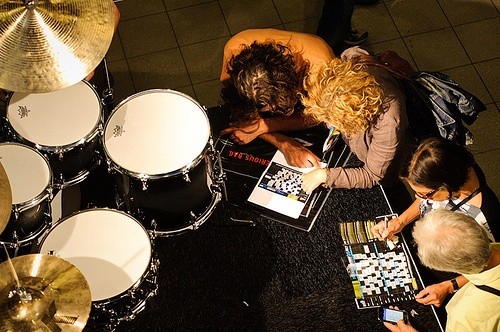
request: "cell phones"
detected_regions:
[377,307,409,325]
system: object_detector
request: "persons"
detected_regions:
[317,0,380,46]
[380,207,500,332]
[219,27,338,169]
[370,136,500,307]
[300,46,410,196]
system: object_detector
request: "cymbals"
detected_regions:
[0,253,91,332]
[0,0,115,95]
[0,161,13,235]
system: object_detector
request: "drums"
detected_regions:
[6,78,105,187]
[101,88,222,237]
[0,142,52,245]
[38,207,161,332]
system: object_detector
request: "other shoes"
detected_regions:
[354,0,380,6]
[343,27,368,44]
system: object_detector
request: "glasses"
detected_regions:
[412,183,449,199]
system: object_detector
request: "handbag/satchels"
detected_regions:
[353,63,489,146]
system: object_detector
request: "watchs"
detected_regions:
[449,278,460,294]
